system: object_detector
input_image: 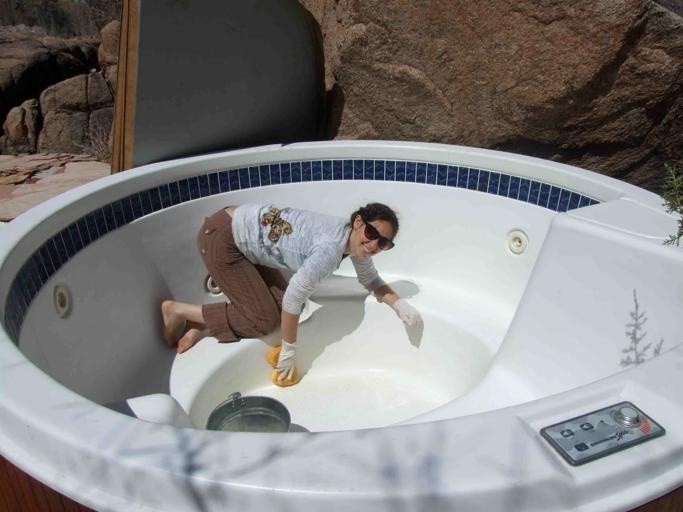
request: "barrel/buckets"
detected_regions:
[205,391,293,432]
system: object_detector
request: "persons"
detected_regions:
[161,202,421,381]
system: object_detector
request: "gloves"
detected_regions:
[277,339,297,381]
[393,298,422,327]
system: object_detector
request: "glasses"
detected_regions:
[361,215,395,250]
